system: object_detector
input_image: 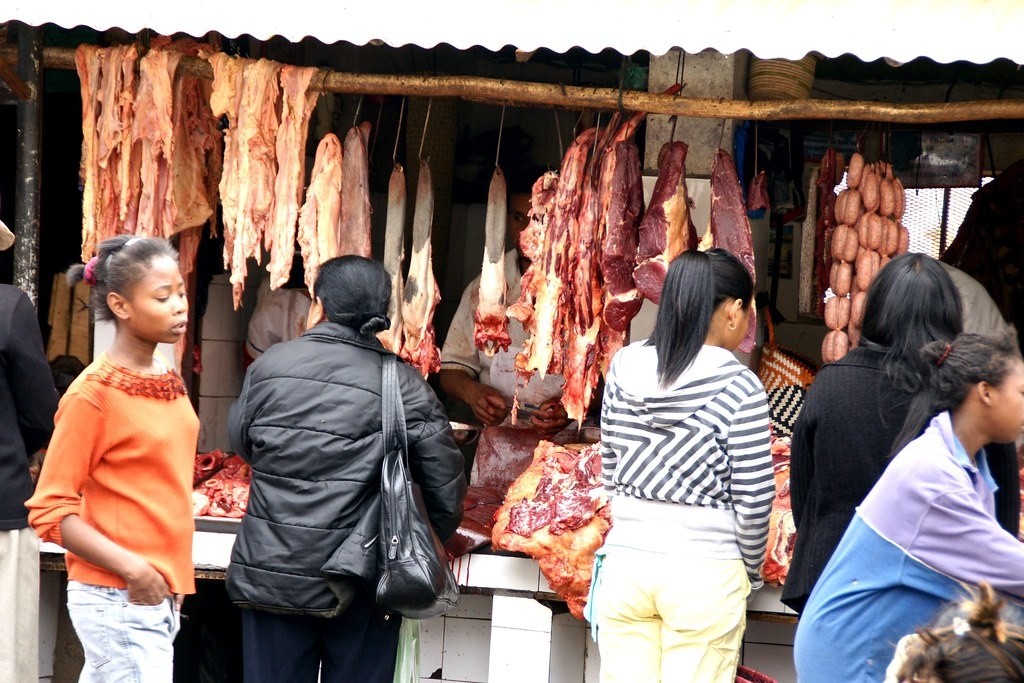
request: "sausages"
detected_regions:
[822,150,908,362]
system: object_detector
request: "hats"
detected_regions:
[0,220,15,252]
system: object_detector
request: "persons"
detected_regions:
[779,253,1020,626]
[0,221,63,683]
[24,235,199,683]
[439,179,591,490]
[227,255,467,683]
[793,331,1023,683]
[583,249,776,683]
[239,270,313,377]
[935,258,1008,336]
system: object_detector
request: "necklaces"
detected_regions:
[115,354,169,376]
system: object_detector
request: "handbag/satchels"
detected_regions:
[372,355,460,618]
